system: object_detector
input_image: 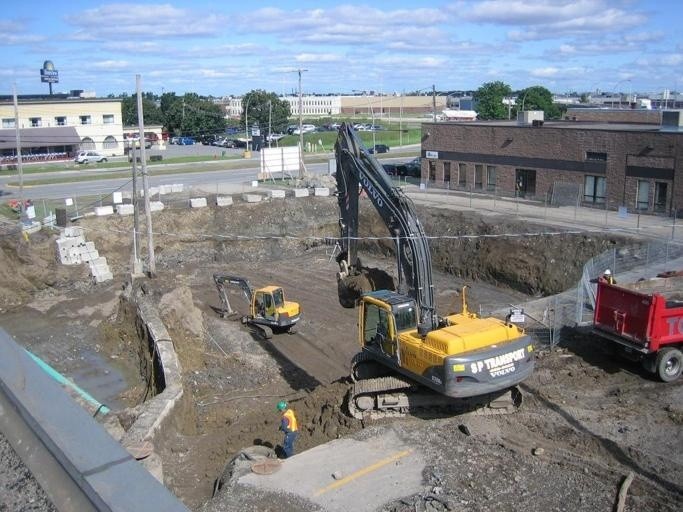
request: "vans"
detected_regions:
[73,149,107,164]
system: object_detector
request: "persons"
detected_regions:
[275,398,298,459]
[24,200,35,221]
[514,177,523,197]
[589,268,618,286]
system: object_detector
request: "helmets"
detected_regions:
[604,269,611,274]
[278,402,286,410]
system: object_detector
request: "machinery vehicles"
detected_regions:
[329,119,540,421]
[210,271,303,339]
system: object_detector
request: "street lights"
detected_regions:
[610,79,631,108]
[289,69,309,148]
[351,82,545,146]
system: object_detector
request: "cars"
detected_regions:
[287,121,383,136]
[161,126,285,150]
[367,143,390,154]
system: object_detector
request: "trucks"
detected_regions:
[586,269,683,386]
